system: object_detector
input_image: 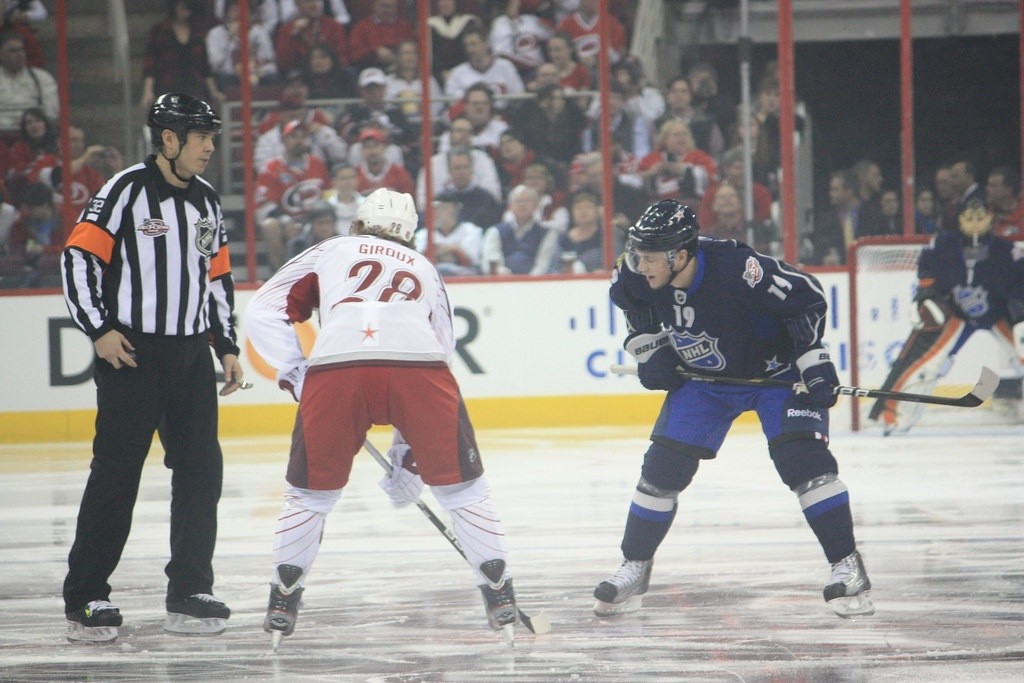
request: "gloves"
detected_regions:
[624,325,693,392]
[795,348,840,410]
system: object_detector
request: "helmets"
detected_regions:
[147,92,222,145]
[356,188,420,242]
[630,199,699,275]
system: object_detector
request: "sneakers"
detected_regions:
[593,559,653,617]
[66,598,123,646]
[162,593,231,637]
[476,558,518,647]
[263,563,305,652]
[824,548,876,619]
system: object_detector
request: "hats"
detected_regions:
[282,119,308,137]
[358,68,388,90]
[361,128,387,142]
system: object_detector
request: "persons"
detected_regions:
[0,0,123,283]
[867,197,1024,436]
[141,0,804,278]
[242,186,521,650]
[813,153,1024,265]
[594,198,875,617]
[62,92,242,641]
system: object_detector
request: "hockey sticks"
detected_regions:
[363,437,553,635]
[609,363,1001,408]
